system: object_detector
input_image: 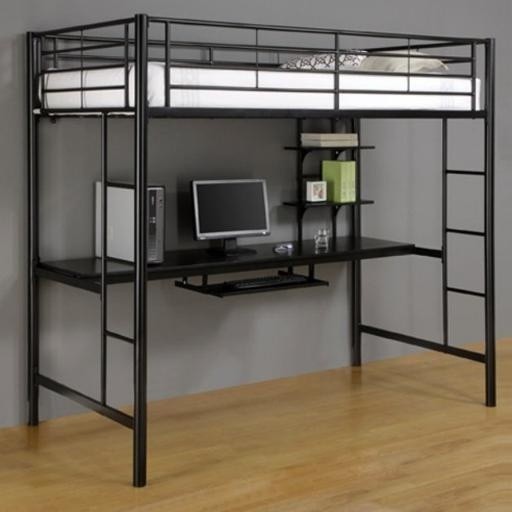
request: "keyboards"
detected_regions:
[224,275,306,290]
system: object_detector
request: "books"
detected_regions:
[321,159,357,204]
[298,132,359,148]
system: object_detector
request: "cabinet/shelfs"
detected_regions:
[283,119,375,235]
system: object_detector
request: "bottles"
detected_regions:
[315,228,328,251]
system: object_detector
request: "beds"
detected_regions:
[29,15,497,489]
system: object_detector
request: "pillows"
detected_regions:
[277,48,367,73]
[361,49,449,75]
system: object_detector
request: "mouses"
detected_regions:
[272,243,293,251]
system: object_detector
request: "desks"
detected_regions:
[40,236,414,297]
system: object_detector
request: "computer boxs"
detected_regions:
[94,180,165,265]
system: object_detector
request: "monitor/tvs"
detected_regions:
[193,179,271,256]
